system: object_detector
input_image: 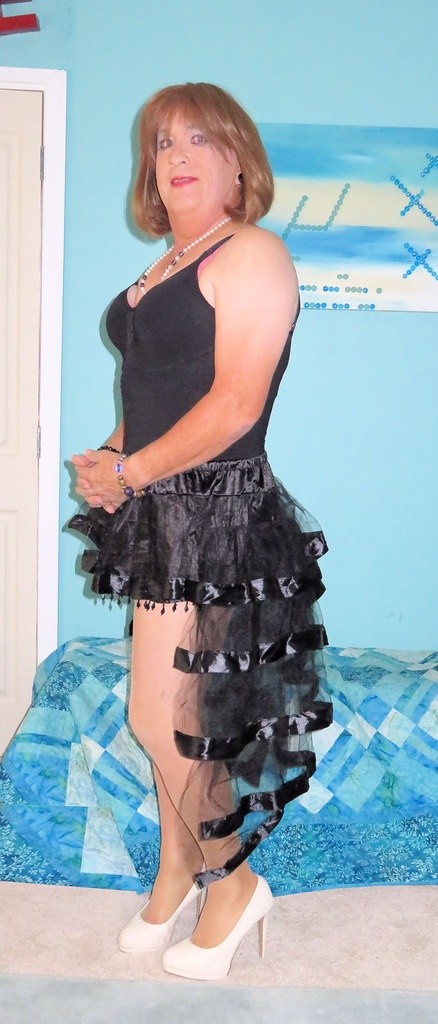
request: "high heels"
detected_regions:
[116,861,208,958]
[162,872,275,982]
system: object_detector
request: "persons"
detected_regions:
[68,81,332,982]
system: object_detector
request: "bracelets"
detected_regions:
[97,446,120,453]
[115,454,145,497]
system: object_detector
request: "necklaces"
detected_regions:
[139,217,233,296]
